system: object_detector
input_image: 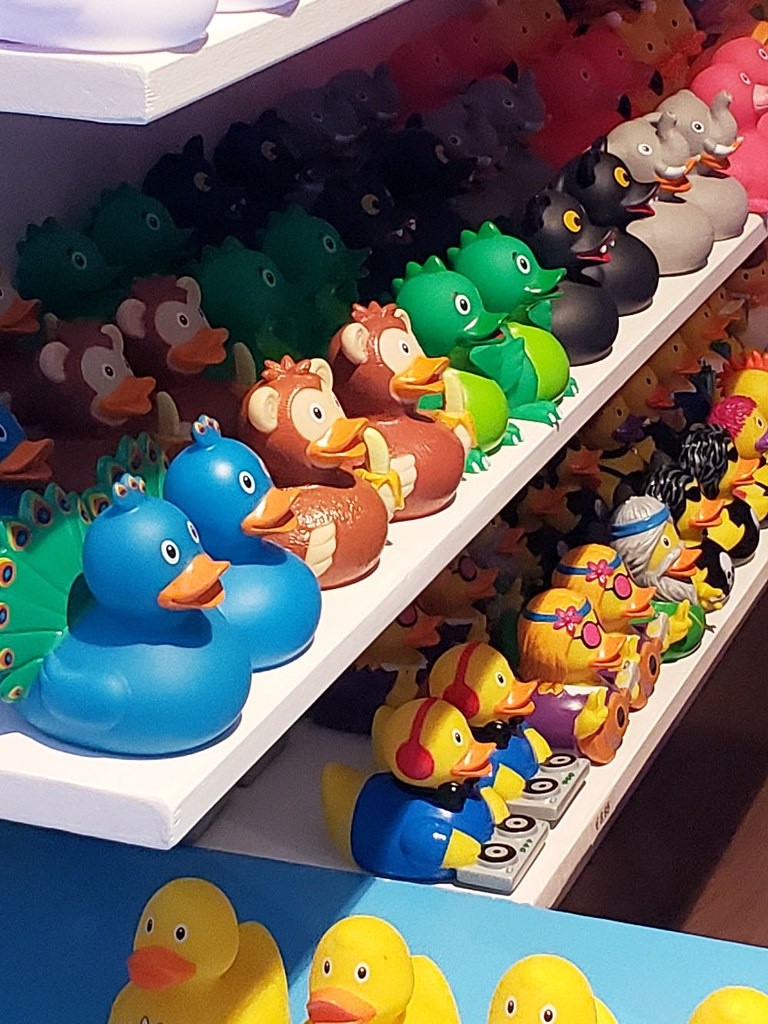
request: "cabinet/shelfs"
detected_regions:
[0,0,768,911]
